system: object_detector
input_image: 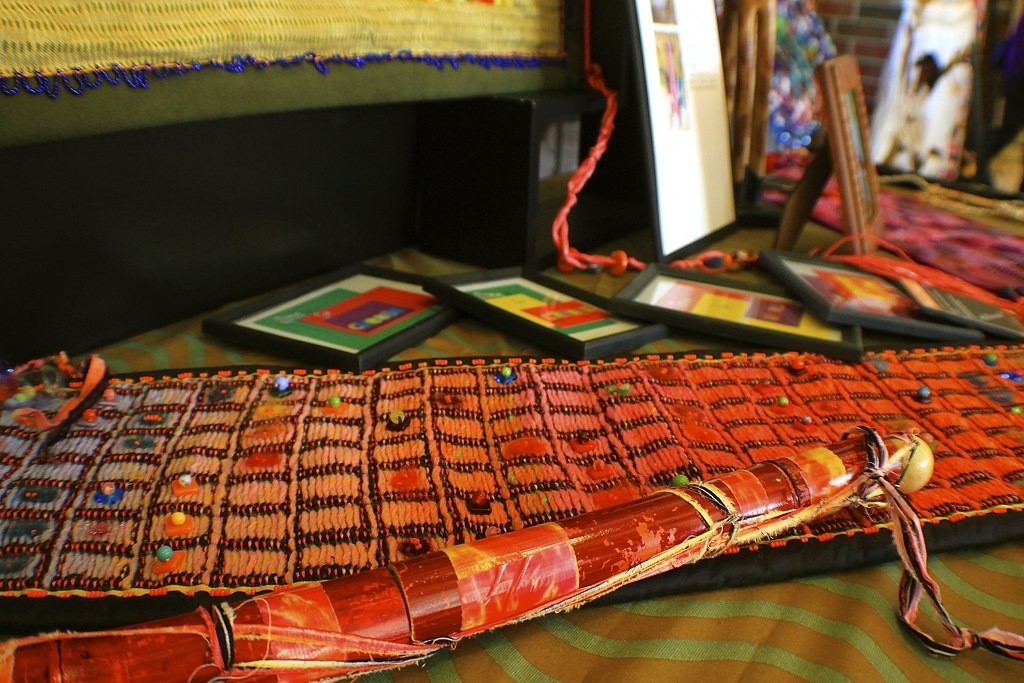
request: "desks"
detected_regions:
[0,187,1024,683]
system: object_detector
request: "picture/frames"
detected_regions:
[813,52,889,256]
[608,262,864,363]
[421,264,673,362]
[200,260,467,376]
[622,0,740,263]
[758,248,985,344]
